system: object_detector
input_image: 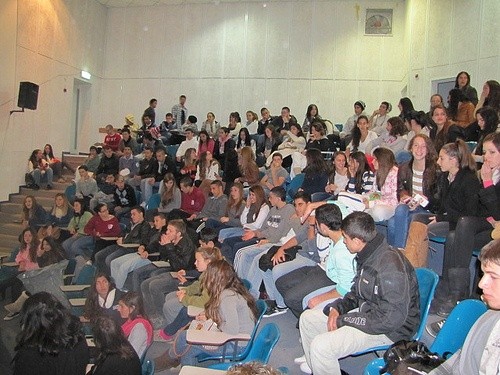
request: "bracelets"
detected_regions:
[307,224,315,229]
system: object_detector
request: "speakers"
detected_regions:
[17,82,39,110]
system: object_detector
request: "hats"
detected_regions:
[354,100,366,110]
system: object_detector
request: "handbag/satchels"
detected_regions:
[25,171,34,188]
[171,318,221,358]
[380,339,454,375]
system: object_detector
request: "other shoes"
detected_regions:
[426,318,446,337]
[3,312,20,320]
[261,306,289,316]
[294,354,312,374]
[152,330,172,341]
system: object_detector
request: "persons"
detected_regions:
[0,71,500,375]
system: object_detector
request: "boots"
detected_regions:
[402,221,430,268]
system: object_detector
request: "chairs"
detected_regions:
[1,124,490,375]
